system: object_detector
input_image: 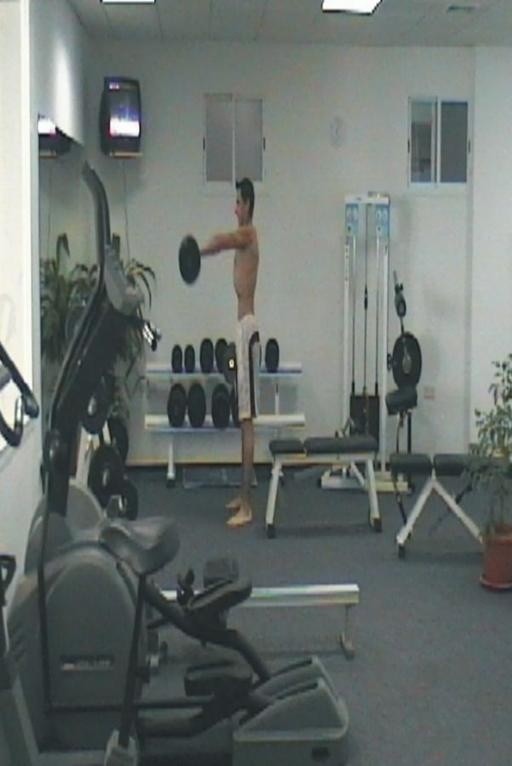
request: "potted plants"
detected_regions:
[460,352,510,594]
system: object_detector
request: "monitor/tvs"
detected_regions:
[99,76,142,155]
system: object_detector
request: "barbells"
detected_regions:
[179,235,200,284]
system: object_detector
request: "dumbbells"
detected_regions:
[167,338,279,430]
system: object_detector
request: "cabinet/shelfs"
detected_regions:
[145,358,309,491]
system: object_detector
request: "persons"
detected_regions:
[201,178,262,526]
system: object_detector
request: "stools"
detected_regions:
[262,436,485,561]
